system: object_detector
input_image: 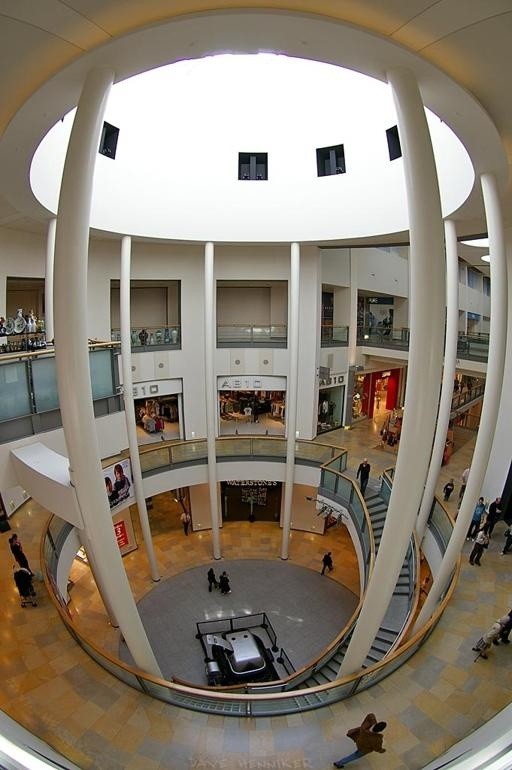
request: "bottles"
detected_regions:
[2,340,18,353]
[27,318,32,333]
[32,319,37,334]
[20,336,46,351]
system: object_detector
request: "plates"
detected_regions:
[2,317,16,335]
[13,316,26,334]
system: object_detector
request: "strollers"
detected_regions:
[11,564,40,608]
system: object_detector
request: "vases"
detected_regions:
[171,327,178,344]
[164,329,169,343]
[26,318,32,332]
[131,331,138,347]
[139,329,147,345]
[156,330,162,344]
[32,320,36,332]
[149,332,155,345]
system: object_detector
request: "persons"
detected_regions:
[356,457,371,499]
[332,713,388,768]
[473,615,510,659]
[219,571,230,595]
[320,552,334,576]
[103,477,118,508]
[9,534,35,577]
[113,464,130,502]
[443,466,512,566]
[208,567,218,592]
[180,510,190,536]
[491,610,512,647]
